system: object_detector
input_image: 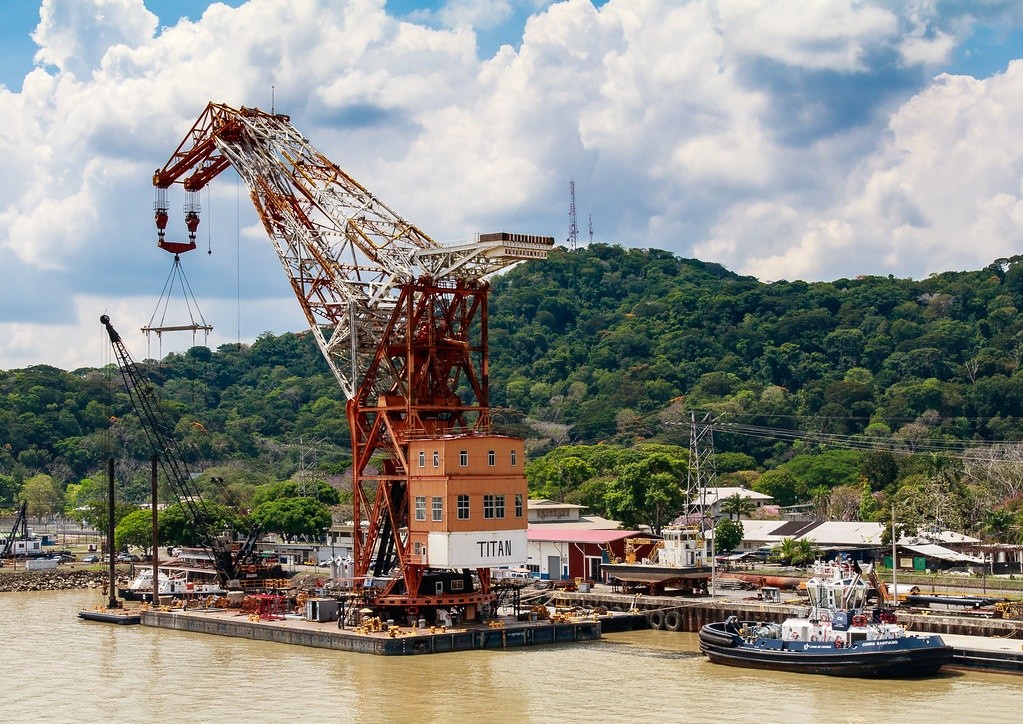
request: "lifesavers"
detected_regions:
[852,614,867,627]
[879,613,894,624]
[821,614,827,622]
[649,610,666,630]
[835,640,841,648]
[791,632,799,639]
[248,565,257,573]
[810,635,818,642]
[664,611,683,631]
[910,586,920,595]
[407,607,419,615]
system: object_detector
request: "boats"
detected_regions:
[698,571,954,680]
[598,561,719,585]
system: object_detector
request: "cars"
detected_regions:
[122,554,140,563]
[51,555,66,566]
[99,552,129,563]
[83,556,99,563]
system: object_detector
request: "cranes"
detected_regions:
[99,315,288,606]
[149,100,560,624]
